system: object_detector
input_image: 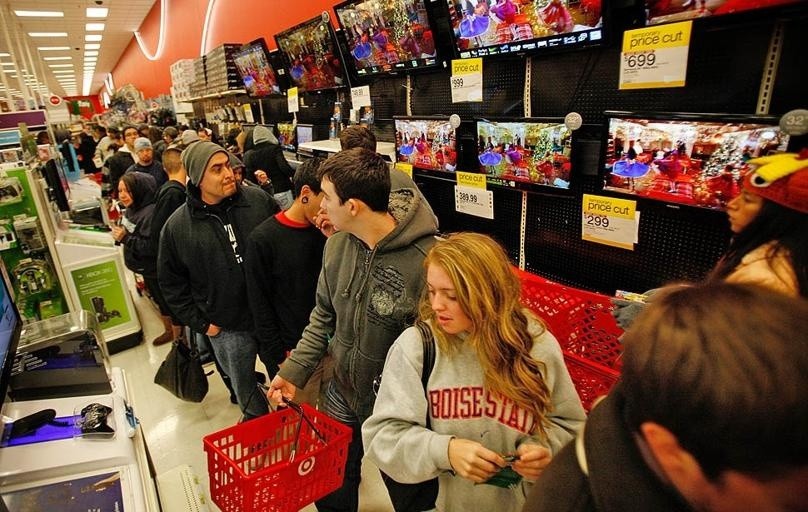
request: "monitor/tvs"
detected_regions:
[640,0,804,28]
[392,116,459,174]
[59,139,74,172]
[0,257,22,410]
[599,111,796,220]
[334,0,443,82]
[274,14,351,95]
[217,104,317,159]
[230,38,284,99]
[472,116,577,199]
[443,0,609,62]
[46,156,71,211]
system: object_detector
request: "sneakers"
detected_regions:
[153,332,173,346]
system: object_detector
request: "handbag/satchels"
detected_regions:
[366,376,444,512]
[155,340,209,402]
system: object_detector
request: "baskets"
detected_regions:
[511,267,645,413]
[202,400,353,511]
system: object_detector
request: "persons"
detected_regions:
[641,150,807,304]
[267,147,441,511]
[520,279,808,512]
[362,232,590,512]
[69,119,332,472]
[341,126,431,212]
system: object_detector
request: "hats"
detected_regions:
[743,151,806,212]
[182,130,199,145]
[134,137,153,153]
[180,140,229,187]
